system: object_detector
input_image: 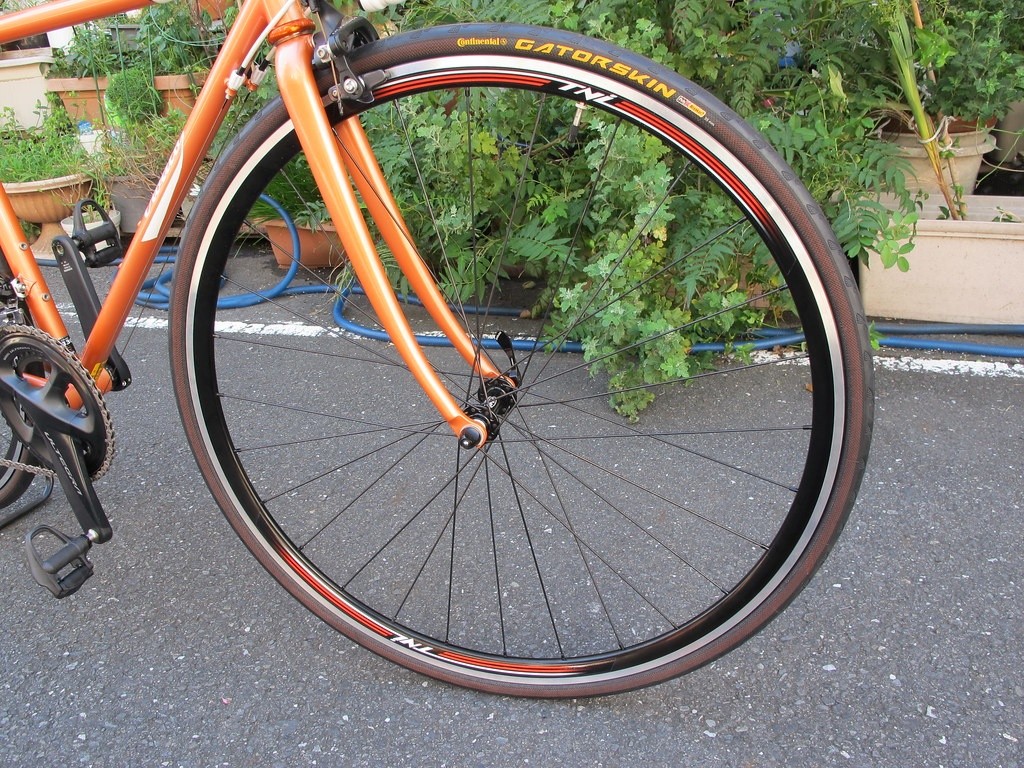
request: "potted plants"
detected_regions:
[0,1,1024,418]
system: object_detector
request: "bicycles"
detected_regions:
[0,1,879,699]
